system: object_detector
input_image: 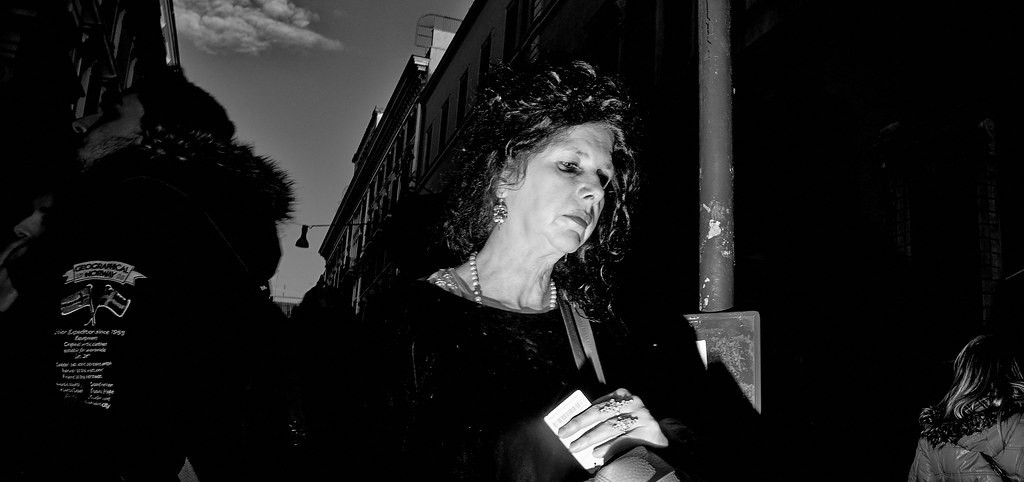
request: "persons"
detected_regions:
[0,52,292,482]
[280,59,775,482]
[907,334,1024,482]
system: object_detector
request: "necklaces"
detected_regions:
[469,250,558,312]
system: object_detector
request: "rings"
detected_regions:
[598,397,634,417]
[612,414,638,435]
